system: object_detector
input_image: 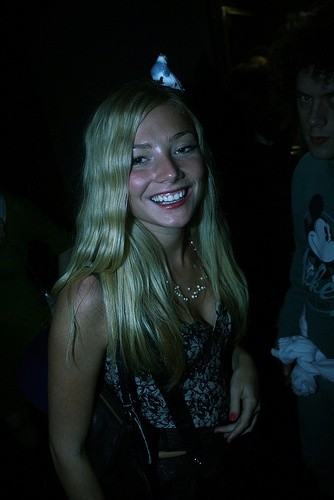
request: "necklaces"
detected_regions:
[163,240,208,303]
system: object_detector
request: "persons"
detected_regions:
[272,32,334,500]
[0,193,75,472]
[43,82,263,499]
